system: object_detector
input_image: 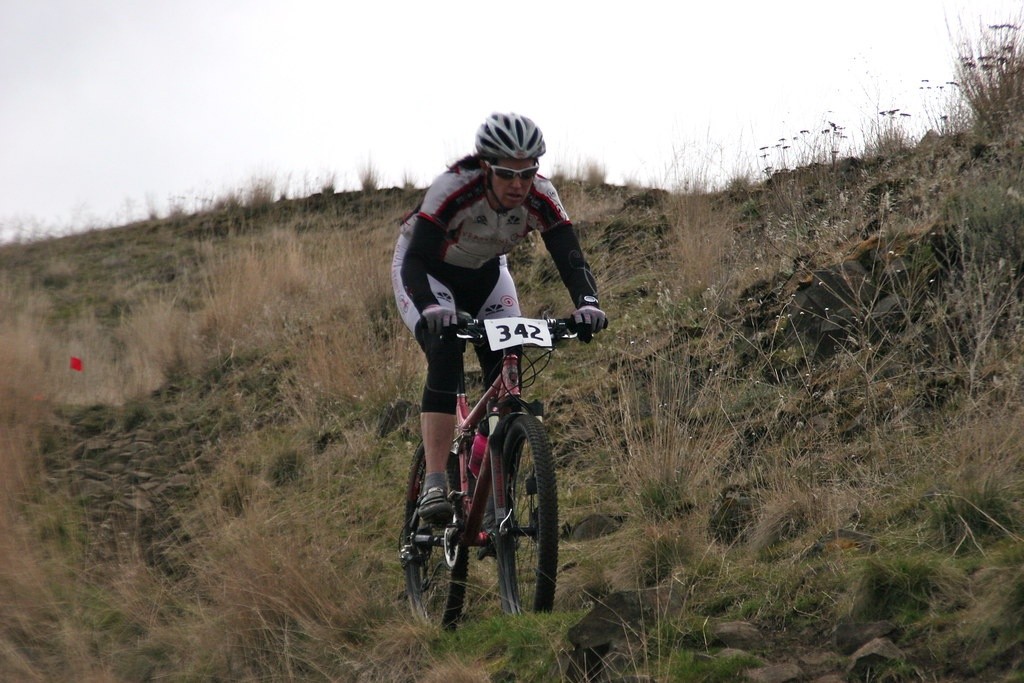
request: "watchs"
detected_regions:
[577,294,600,308]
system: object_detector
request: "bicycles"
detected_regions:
[396,311,612,632]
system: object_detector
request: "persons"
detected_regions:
[390,112,607,556]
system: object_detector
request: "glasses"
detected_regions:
[483,159,540,179]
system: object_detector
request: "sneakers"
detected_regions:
[479,511,523,553]
[420,488,453,524]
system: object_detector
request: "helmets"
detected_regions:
[475,111,546,160]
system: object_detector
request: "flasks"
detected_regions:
[468,419,489,478]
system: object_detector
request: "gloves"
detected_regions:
[421,307,456,335]
[571,307,606,335]
